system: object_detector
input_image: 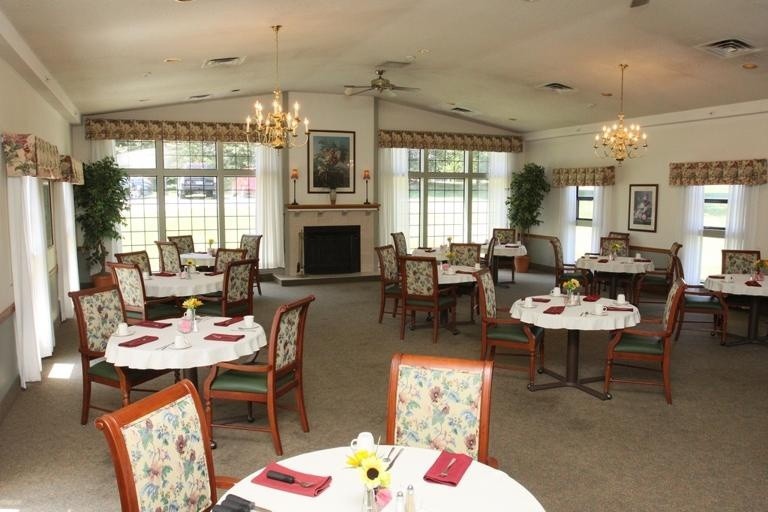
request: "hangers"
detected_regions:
[383,442,397,462]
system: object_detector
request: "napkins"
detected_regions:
[605,306,634,312]
[216,318,244,328]
[583,294,601,302]
[131,318,173,329]
[544,306,565,314]
[118,336,159,349]
[532,297,551,303]
[203,333,246,342]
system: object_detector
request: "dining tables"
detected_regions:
[74,156,130,287]
[505,163,550,272]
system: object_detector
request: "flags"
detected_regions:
[330,189,336,207]
[363,483,377,512]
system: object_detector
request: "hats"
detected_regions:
[118,177,152,200]
[232,175,257,198]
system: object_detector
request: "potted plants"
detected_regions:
[628,184,658,233]
[505,163,550,272]
[307,129,355,193]
[74,156,130,287]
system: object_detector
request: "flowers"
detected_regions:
[346,452,391,489]
[318,148,347,189]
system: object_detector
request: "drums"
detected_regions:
[384,446,405,470]
[207,491,271,512]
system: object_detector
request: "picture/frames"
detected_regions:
[307,129,355,193]
[628,184,658,233]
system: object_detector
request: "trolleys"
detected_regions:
[343,70,420,96]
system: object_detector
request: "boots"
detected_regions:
[177,163,216,199]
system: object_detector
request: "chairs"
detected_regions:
[105,236,262,318]
[473,266,546,388]
[69,285,181,427]
[386,352,497,467]
[203,296,316,456]
[375,228,768,346]
[604,278,687,404]
[94,379,244,512]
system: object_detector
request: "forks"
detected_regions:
[437,457,460,477]
[265,469,316,489]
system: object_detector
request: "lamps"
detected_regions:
[245,26,310,149]
[291,169,298,205]
[363,170,371,204]
[593,64,647,168]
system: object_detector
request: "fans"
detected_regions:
[343,70,420,96]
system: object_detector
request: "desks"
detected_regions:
[109,316,264,396]
[515,294,639,401]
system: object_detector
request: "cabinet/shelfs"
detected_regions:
[393,483,417,512]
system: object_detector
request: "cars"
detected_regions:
[174,334,188,347]
[595,304,606,314]
[244,313,255,328]
[524,296,533,306]
[551,286,561,295]
[143,271,149,279]
[349,429,376,456]
[117,322,129,334]
[626,251,642,264]
[617,294,626,304]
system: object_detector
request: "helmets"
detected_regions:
[110,331,136,336]
[238,324,259,330]
[168,345,194,349]
[590,311,609,317]
[518,302,536,308]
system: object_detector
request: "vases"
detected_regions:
[330,189,336,207]
[363,483,377,512]
[346,452,391,489]
[318,148,347,189]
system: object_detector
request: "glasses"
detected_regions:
[593,64,647,168]
[363,170,371,204]
[245,26,310,149]
[291,169,298,205]
